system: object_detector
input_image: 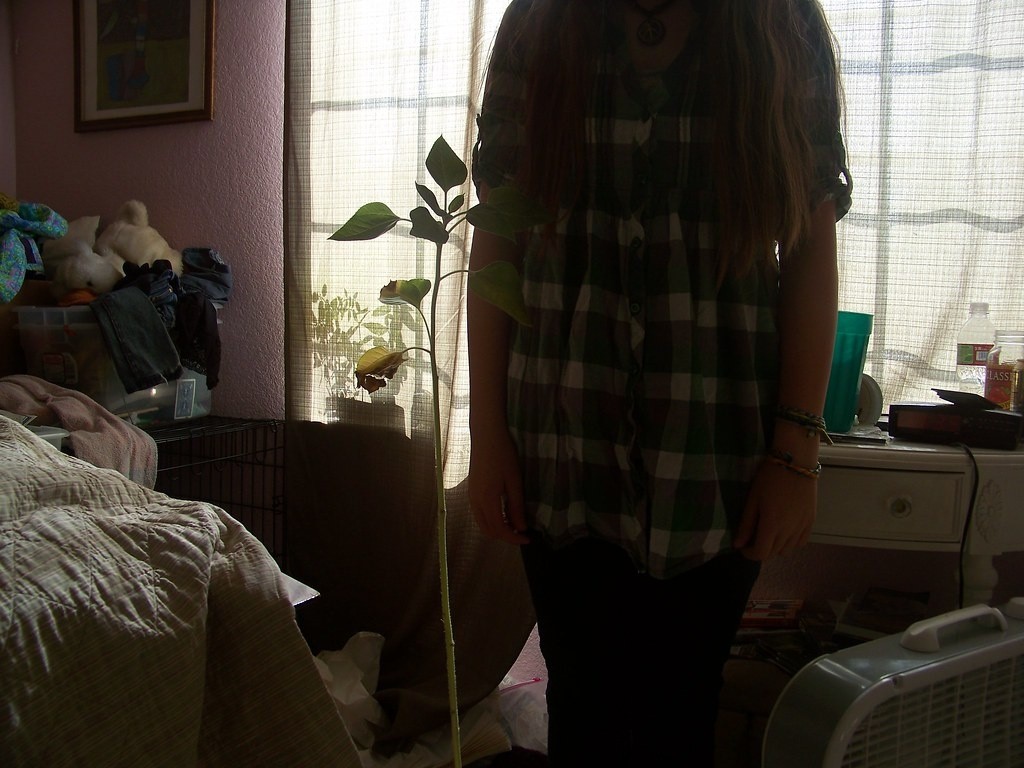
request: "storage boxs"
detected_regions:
[11,301,225,427]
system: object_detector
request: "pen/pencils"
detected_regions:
[826,434,886,444]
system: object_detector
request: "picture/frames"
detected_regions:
[71,0,218,133]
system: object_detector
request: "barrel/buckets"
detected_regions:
[825,311,873,433]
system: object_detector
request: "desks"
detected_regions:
[807,431,1024,609]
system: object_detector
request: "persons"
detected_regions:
[465,0,853,768]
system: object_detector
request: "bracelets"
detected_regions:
[772,403,836,445]
[773,450,825,477]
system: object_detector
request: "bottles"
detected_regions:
[956,302,996,397]
[984,331,1024,414]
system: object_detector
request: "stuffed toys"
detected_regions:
[42,199,184,308]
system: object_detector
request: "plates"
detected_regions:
[857,374,883,427]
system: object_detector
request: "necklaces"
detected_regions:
[625,0,672,46]
